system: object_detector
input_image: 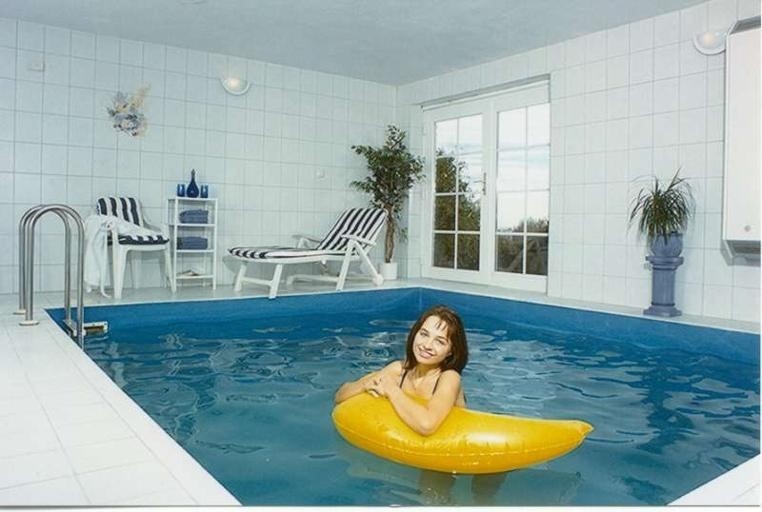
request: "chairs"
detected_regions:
[228,206,387,301]
[96,197,178,303]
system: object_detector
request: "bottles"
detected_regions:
[186,169,199,197]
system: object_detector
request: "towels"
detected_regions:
[177,209,208,250]
[81,215,163,294]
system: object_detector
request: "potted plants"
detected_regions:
[624,164,697,258]
[349,123,428,280]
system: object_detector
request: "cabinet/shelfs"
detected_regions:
[164,196,218,294]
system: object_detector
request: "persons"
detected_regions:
[334,305,467,437]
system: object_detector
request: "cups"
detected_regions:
[177,184,185,196]
[200,185,208,198]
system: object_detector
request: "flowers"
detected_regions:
[103,82,152,138]
